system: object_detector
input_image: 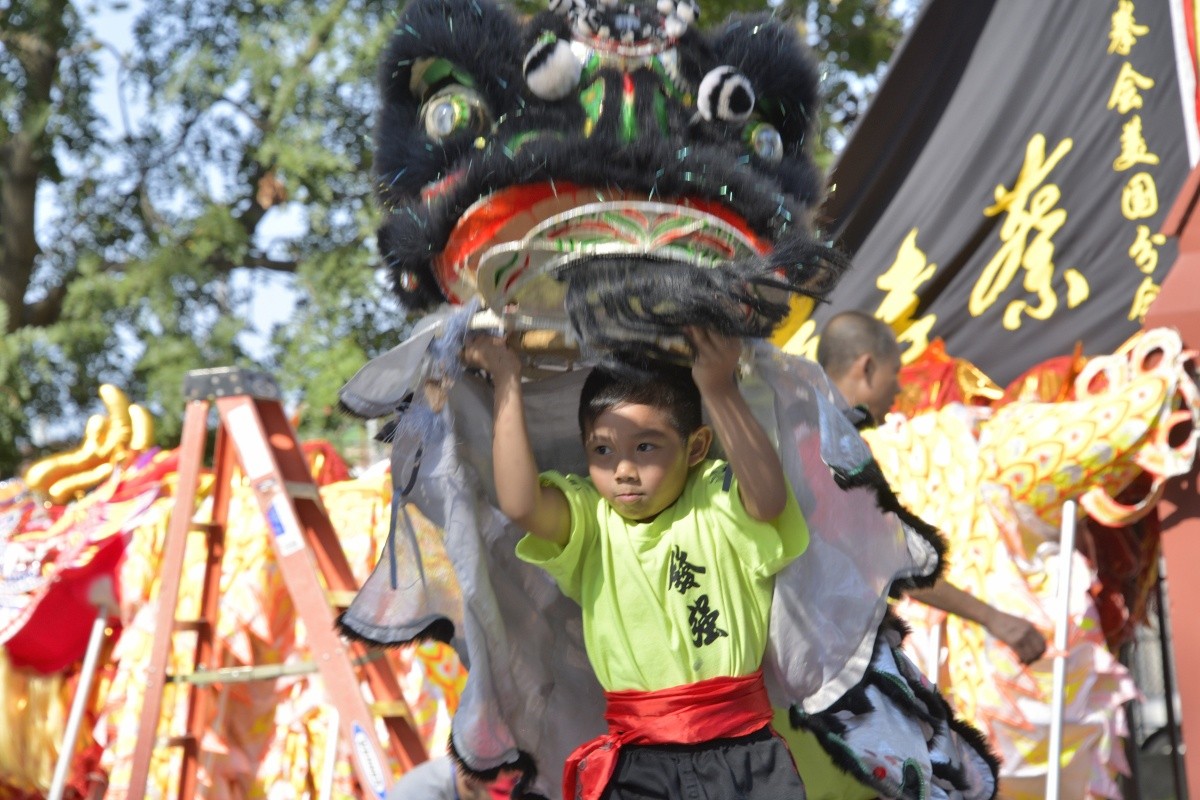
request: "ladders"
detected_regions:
[108,367,431,799]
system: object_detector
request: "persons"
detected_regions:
[773,314,1045,797]
[466,312,814,800]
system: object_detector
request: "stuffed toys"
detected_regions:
[368,1,821,344]
